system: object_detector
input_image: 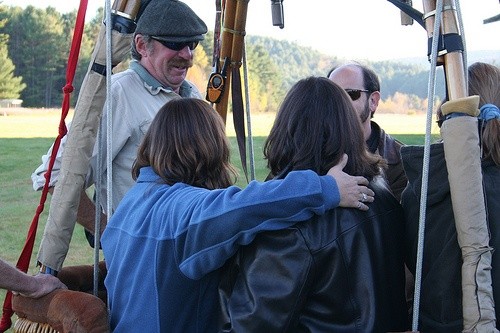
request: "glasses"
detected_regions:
[344,89,369,101]
[154,41,198,50]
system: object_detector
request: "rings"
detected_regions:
[356,204,362,210]
[360,193,369,201]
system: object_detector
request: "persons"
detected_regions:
[30,0,207,253]
[0,259,68,298]
[98,63,500,333]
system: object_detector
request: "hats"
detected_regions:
[135,0,208,41]
